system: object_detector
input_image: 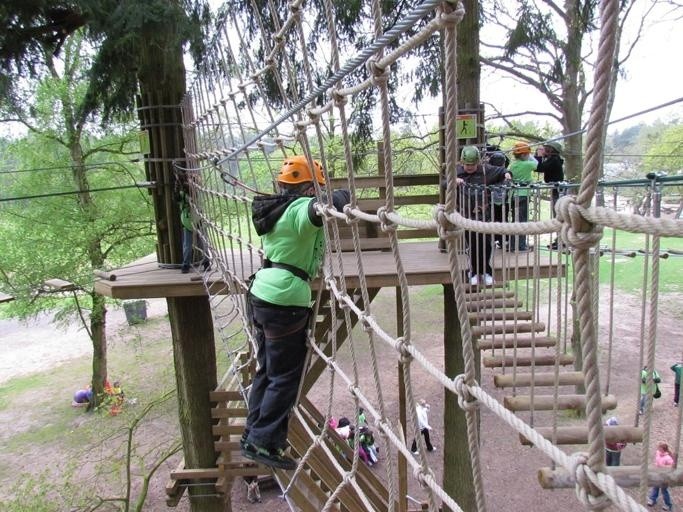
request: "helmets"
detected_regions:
[489,153,506,165]
[461,145,480,165]
[512,143,531,154]
[277,156,326,186]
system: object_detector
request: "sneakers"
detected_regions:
[468,271,496,286]
[548,242,565,251]
[648,501,655,506]
[663,505,669,511]
[239,430,298,471]
[505,244,534,253]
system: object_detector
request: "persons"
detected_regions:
[604,417,626,466]
[242,464,262,504]
[240,156,352,470]
[639,366,661,415]
[455,140,566,285]
[325,407,379,466]
[670,360,683,407]
[74,378,124,405]
[648,441,675,510]
[412,399,437,456]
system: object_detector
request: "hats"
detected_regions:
[607,418,619,425]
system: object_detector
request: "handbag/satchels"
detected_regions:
[654,389,661,398]
